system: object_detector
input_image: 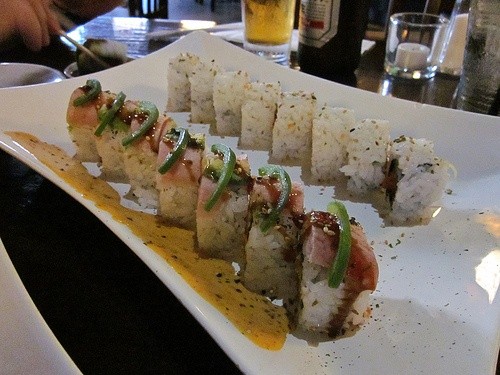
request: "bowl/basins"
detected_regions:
[0,63,65,88]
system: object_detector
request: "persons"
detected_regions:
[0,0,63,49]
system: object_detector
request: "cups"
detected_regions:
[242,0,296,70]
[436,0,472,76]
[384,0,463,85]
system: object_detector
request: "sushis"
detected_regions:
[67,80,379,338]
[75,38,129,74]
[167,52,458,225]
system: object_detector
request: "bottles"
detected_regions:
[298,0,370,79]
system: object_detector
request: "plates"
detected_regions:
[63,56,137,78]
[0,29,500,375]
